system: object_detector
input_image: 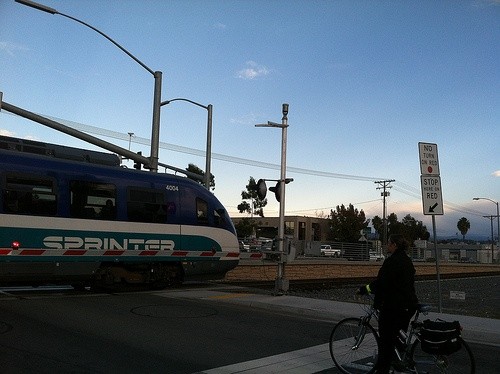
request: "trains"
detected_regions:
[0,130,241,293]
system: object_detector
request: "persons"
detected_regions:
[356,235,417,374]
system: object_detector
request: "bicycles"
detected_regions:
[328,287,477,374]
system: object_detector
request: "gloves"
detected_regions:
[356,284,373,296]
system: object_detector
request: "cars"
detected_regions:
[239,239,385,261]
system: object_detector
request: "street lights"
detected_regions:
[160,97,213,191]
[472,197,500,246]
